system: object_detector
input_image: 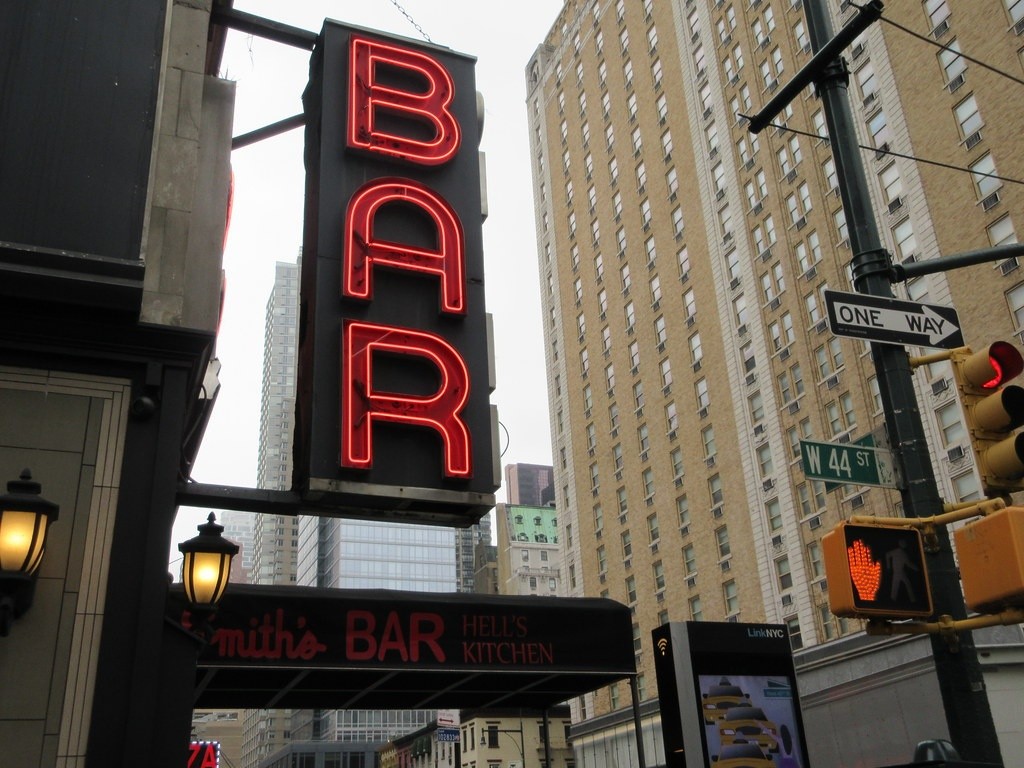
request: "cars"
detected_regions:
[712,748,776,768]
[701,683,754,726]
[717,706,781,753]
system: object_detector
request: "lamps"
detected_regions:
[179,515,239,640]
[0,468,61,636]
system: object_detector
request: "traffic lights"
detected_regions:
[820,521,936,618]
[948,341,1024,497]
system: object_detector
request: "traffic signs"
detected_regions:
[821,288,965,350]
